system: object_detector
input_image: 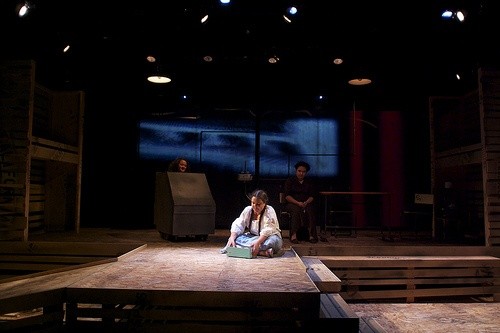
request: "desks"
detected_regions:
[318,192,392,237]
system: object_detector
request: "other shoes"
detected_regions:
[265,247,273,257]
[290,236,299,243]
[305,237,317,242]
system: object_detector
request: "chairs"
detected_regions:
[279,193,308,239]
[400,194,434,239]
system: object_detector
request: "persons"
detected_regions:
[281,161,318,243]
[168,157,194,173]
[221,190,284,258]
